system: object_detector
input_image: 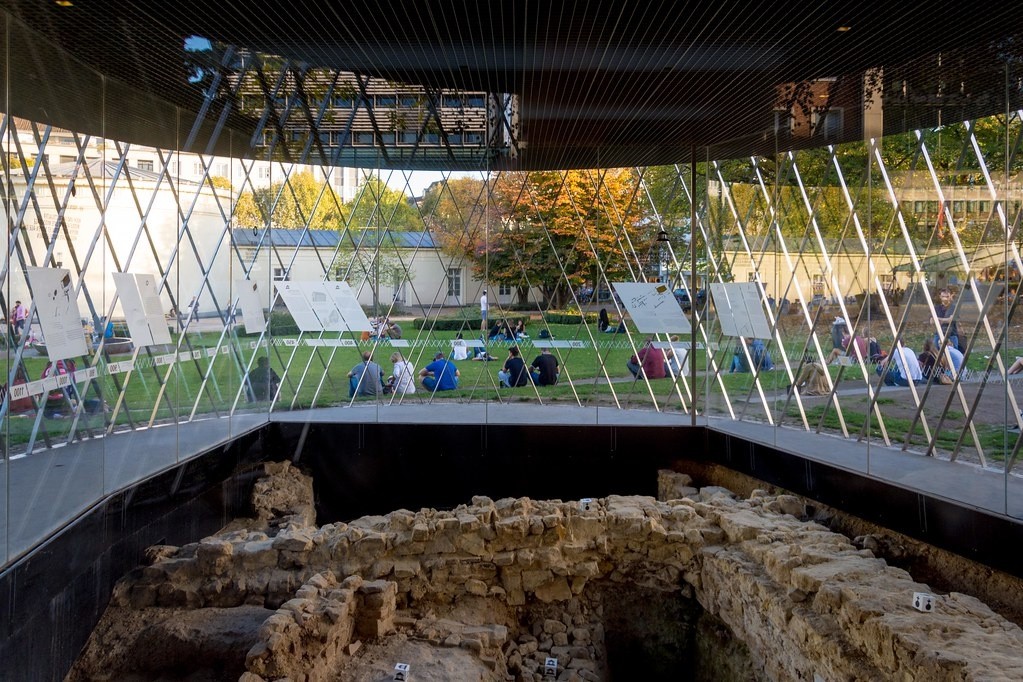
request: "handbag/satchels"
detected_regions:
[958,324,967,353]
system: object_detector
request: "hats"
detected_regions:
[388,319,395,324]
[433,350,441,361]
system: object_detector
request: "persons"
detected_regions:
[419,352,460,392]
[472,333,498,361]
[488,318,525,342]
[498,345,527,390]
[224,300,236,324]
[826,288,968,387]
[0,366,40,416]
[188,296,199,322]
[786,355,829,396]
[627,338,664,379]
[664,335,689,378]
[480,290,489,332]
[81,319,94,343]
[527,347,560,386]
[244,356,281,402]
[729,337,772,372]
[15,301,24,336]
[597,308,634,334]
[361,315,401,341]
[386,352,415,395]
[101,316,115,339]
[348,352,385,398]
[168,308,176,318]
[1006,356,1023,375]
[450,332,472,360]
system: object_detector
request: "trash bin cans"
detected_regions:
[832,322,847,351]
